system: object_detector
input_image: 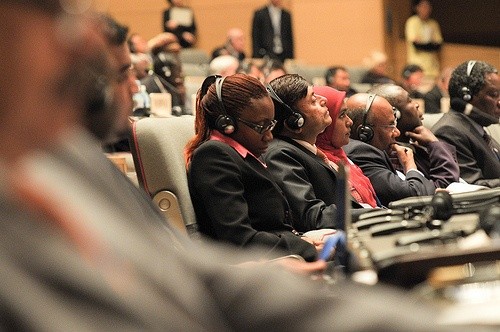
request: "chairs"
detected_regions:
[128,49,500,291]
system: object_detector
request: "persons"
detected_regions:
[207,55,287,86]
[405,0,443,79]
[251,1,295,65]
[39,13,326,286]
[325,66,358,98]
[162,0,197,48]
[258,73,383,233]
[128,34,150,53]
[0,0,500,332]
[360,52,397,84]
[343,92,435,208]
[397,64,457,114]
[366,83,460,188]
[184,73,345,285]
[103,51,193,153]
[148,32,181,59]
[429,59,500,189]
[313,85,389,210]
[208,28,246,64]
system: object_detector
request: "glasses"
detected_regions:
[381,118,398,128]
[235,117,278,134]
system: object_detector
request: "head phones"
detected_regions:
[215,76,238,136]
[158,53,171,77]
[393,107,401,121]
[403,65,413,77]
[356,93,377,142]
[128,40,134,52]
[327,69,334,83]
[423,190,453,229]
[458,61,476,102]
[266,84,306,130]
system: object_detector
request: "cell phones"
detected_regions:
[318,237,339,261]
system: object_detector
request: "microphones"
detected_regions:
[451,97,496,123]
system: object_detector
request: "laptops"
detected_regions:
[336,161,351,272]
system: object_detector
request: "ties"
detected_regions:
[317,148,329,167]
[483,130,496,159]
